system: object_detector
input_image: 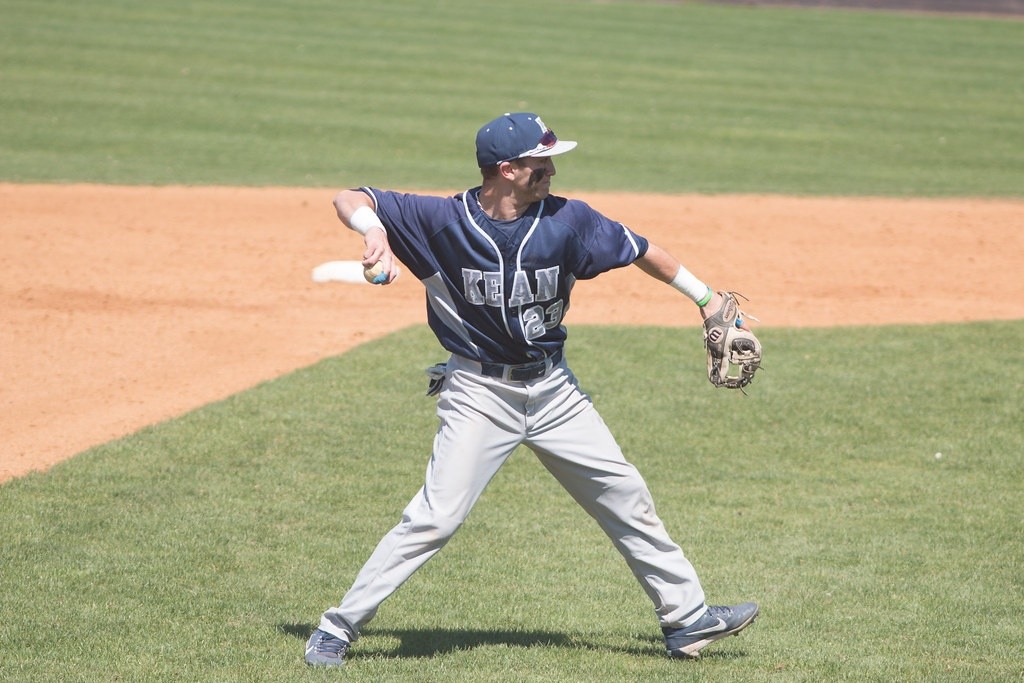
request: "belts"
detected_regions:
[480,349,562,380]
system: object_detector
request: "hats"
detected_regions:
[475,111,578,166]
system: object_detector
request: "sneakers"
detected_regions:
[304,626,350,666]
[665,602,758,655]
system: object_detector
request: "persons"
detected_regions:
[301,113,764,664]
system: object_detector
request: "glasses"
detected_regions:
[497,127,557,165]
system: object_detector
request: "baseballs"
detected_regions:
[363,263,382,284]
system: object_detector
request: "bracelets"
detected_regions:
[697,286,713,308]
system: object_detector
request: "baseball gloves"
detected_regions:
[703,292,762,388]
